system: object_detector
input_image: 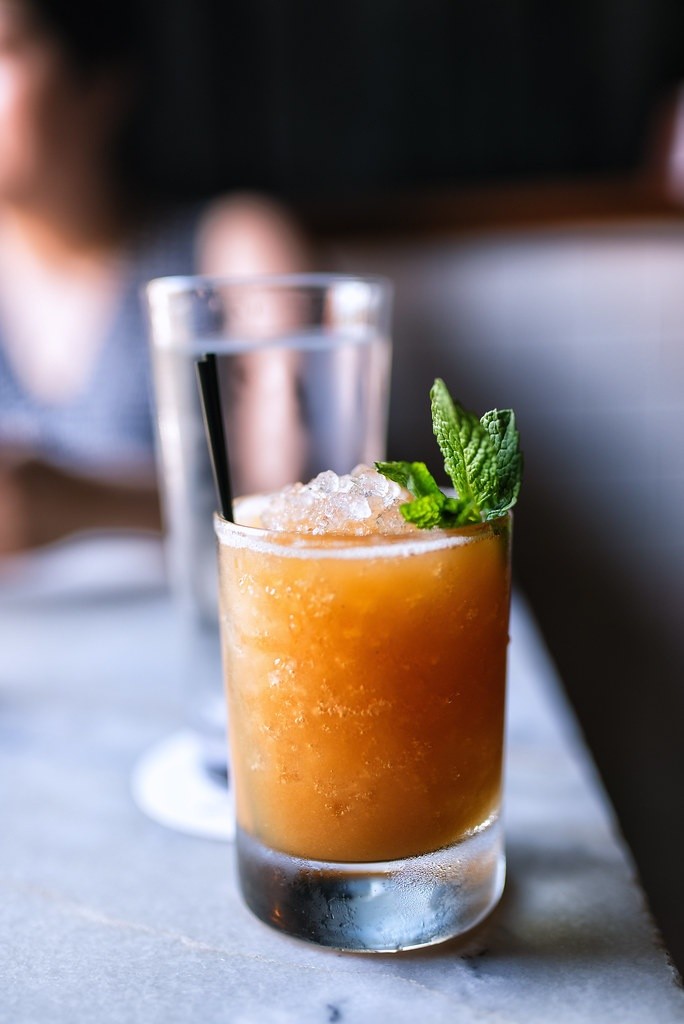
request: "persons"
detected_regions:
[0,0,327,565]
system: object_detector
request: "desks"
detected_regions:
[4,487,684,1019]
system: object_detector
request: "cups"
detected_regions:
[147,273,394,814]
[213,488,510,954]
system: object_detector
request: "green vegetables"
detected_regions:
[370,377,525,530]
